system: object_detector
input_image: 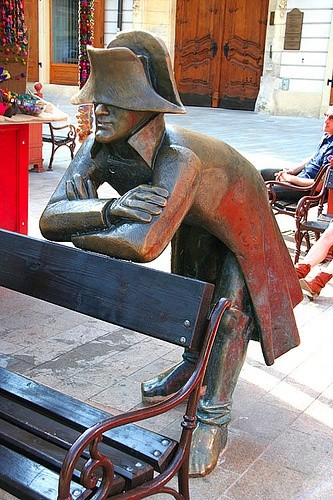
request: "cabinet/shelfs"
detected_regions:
[0,123,44,236]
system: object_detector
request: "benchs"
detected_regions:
[0,229,232,500]
[43,122,78,171]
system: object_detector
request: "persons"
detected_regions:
[259,105,332,200]
[40,32,303,477]
[295,222,333,301]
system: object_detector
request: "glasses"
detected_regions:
[324,113,333,119]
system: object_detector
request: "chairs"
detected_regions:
[265,162,333,263]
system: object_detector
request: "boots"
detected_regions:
[295,263,311,279]
[299,269,333,301]
